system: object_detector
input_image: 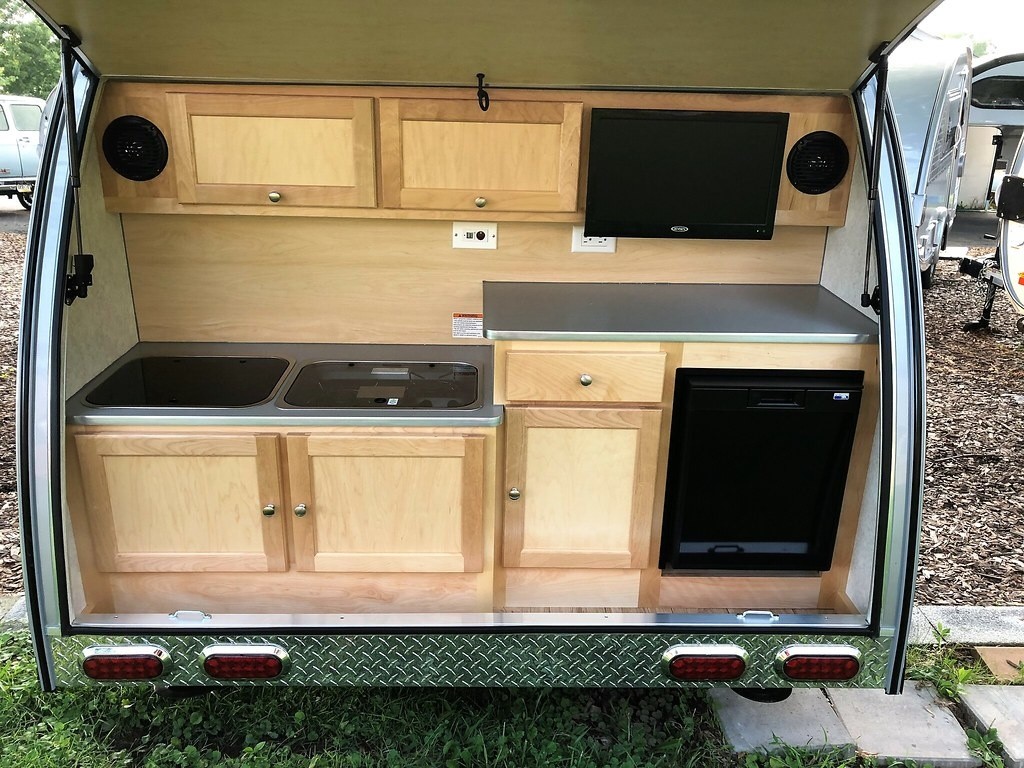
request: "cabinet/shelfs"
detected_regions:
[495,341,677,608]
[167,89,582,213]
[65,424,503,614]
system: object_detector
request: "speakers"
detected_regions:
[102,115,168,182]
[785,130,850,195]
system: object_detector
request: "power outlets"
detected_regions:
[571,226,617,253]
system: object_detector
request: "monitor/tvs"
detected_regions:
[584,108,790,240]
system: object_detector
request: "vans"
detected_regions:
[885,26,1024,335]
[0,0,940,699]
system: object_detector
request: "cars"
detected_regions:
[0,94,46,210]
